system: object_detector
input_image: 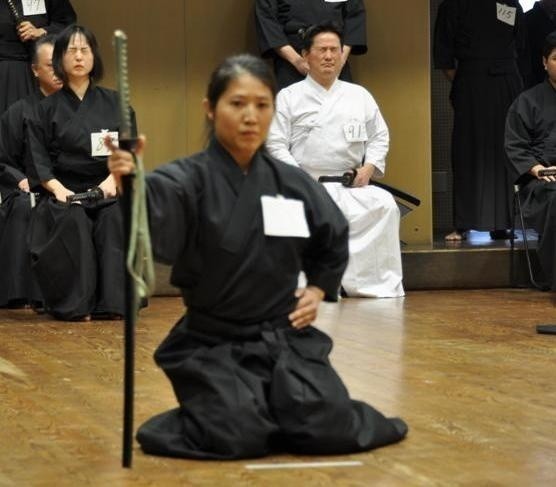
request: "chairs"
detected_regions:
[509,185,551,291]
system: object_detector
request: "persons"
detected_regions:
[430,0,556,240]
[504,32,556,252]
[264,21,406,299]
[255,0,369,90]
[103,52,407,460]
[0,0,139,323]
